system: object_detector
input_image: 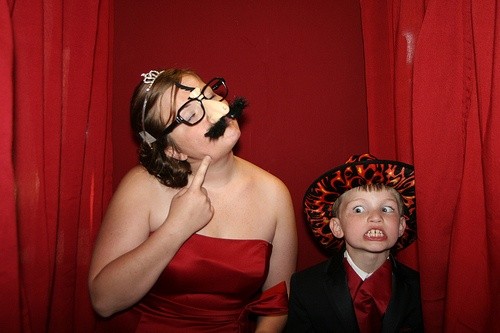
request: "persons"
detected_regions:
[88,64,299,332]
[282,153,425,333]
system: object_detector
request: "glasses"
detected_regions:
[160,76,230,135]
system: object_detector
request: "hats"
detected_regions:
[300,152,419,257]
